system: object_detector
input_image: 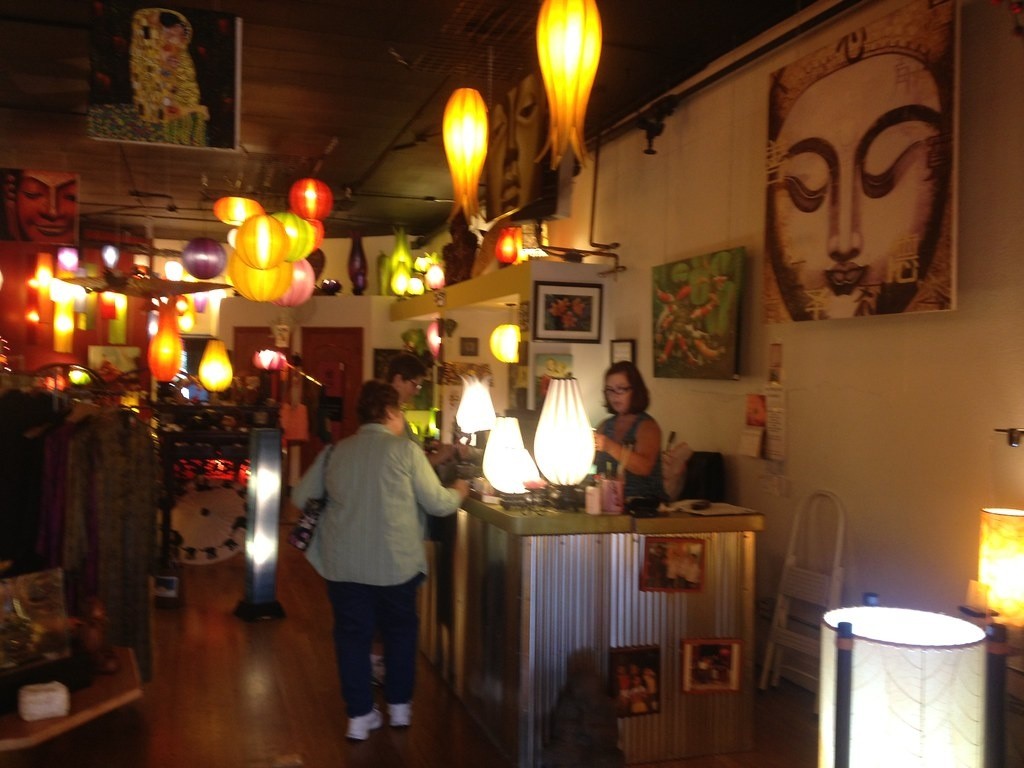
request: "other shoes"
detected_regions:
[371,676,385,687]
[388,703,412,726]
[346,702,383,740]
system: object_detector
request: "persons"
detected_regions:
[590,361,664,508]
[290,381,471,742]
[389,352,456,472]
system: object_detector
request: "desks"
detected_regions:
[0,645,144,751]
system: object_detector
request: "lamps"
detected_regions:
[26,0,603,509]
[818,509,1024,767]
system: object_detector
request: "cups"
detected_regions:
[601,480,624,515]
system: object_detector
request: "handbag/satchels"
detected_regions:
[285,497,327,550]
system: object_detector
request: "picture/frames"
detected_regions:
[609,645,661,718]
[680,638,743,695]
[610,339,636,366]
[638,537,706,593]
[534,281,603,343]
[461,337,478,355]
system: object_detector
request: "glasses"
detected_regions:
[409,380,423,390]
[604,386,633,396]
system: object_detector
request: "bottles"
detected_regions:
[585,481,601,515]
